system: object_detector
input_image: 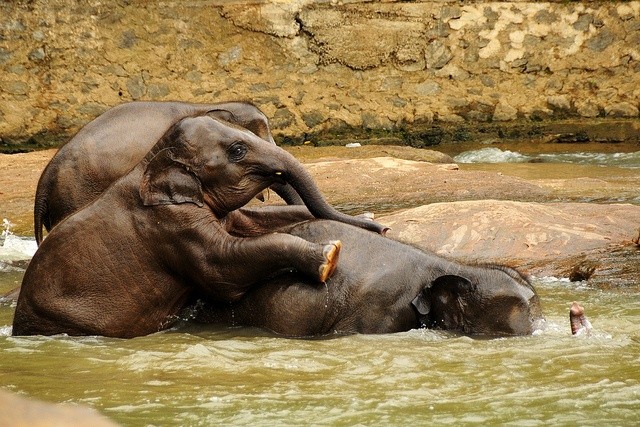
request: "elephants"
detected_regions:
[34,100,306,246]
[11,112,390,338]
[196,217,594,338]
